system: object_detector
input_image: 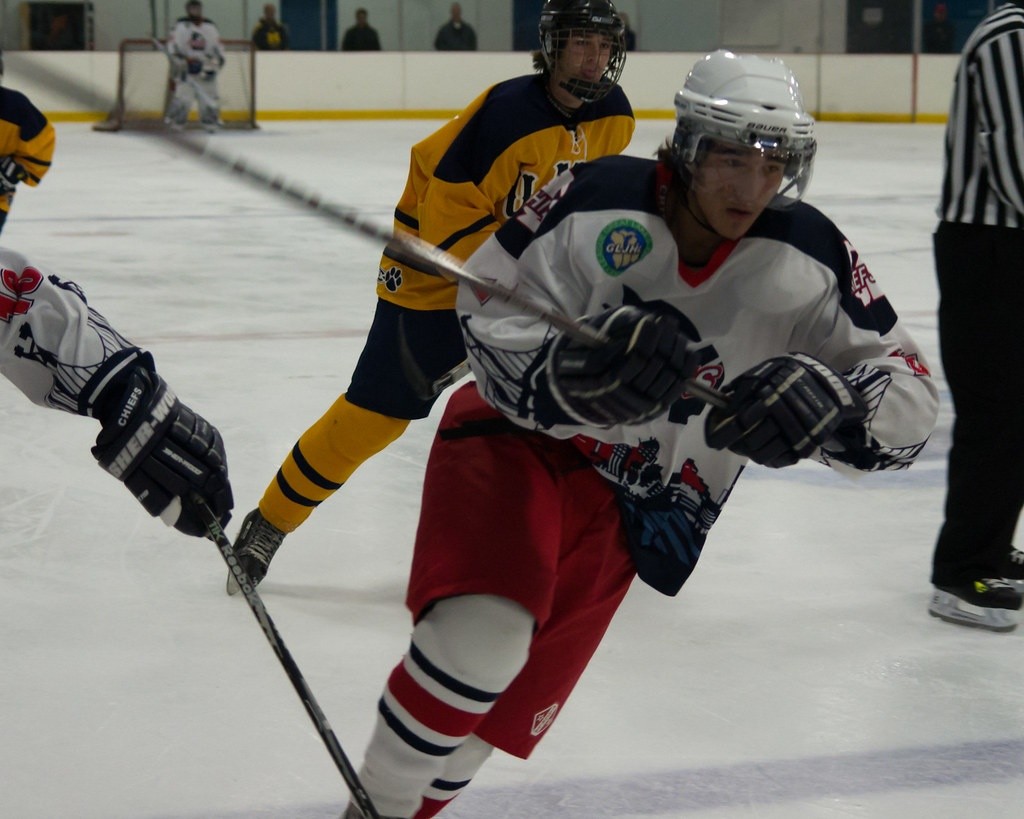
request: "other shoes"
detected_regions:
[198,103,225,131]
[160,106,188,134]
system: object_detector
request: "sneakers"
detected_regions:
[231,505,283,593]
[923,542,1023,633]
[224,508,284,594]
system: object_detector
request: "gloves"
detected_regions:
[88,344,234,541]
[172,51,220,83]
[543,300,695,431]
[705,353,860,469]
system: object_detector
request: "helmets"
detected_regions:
[535,1,628,101]
[675,53,816,211]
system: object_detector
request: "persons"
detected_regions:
[434,2,477,51]
[227,1,634,597]
[928,1,1023,629]
[251,3,290,51]
[925,2,957,54]
[620,11,636,52]
[0,237,235,540]
[166,0,226,132]
[0,45,56,238]
[342,7,382,52]
[35,13,82,50]
[336,54,940,819]
